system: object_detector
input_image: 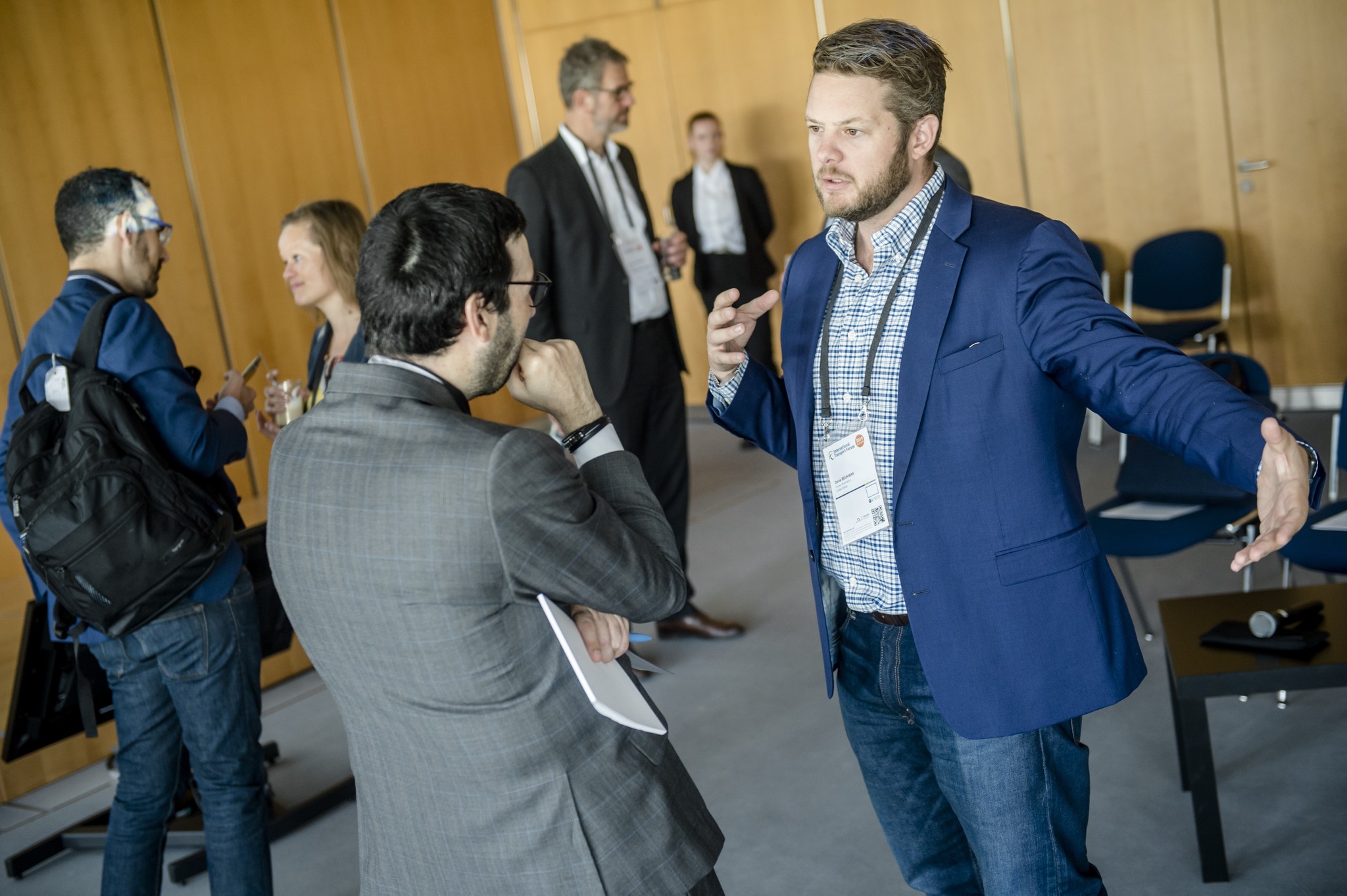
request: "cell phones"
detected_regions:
[219,355,261,402]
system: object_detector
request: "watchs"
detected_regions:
[1300,443,1317,483]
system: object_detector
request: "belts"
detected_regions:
[869,611,909,626]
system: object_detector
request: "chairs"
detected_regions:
[1272,380,1347,712]
[1071,234,1115,449]
[1113,224,1235,474]
[1149,582,1347,884]
[1083,348,1274,637]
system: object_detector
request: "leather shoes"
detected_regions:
[657,603,746,640]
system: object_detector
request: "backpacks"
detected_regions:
[6,293,235,640]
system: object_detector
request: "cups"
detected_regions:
[273,379,305,429]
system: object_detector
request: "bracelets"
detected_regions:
[561,414,612,455]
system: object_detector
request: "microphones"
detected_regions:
[1248,597,1326,638]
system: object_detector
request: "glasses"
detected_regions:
[131,214,172,245]
[508,270,551,308]
[590,81,632,95]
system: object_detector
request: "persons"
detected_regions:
[0,165,279,896]
[702,16,1323,896]
[225,182,728,895]
[499,38,746,644]
[667,113,777,452]
[249,198,379,443]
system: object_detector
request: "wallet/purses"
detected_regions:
[1200,620,1332,664]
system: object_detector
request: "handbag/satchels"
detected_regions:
[237,520,293,658]
